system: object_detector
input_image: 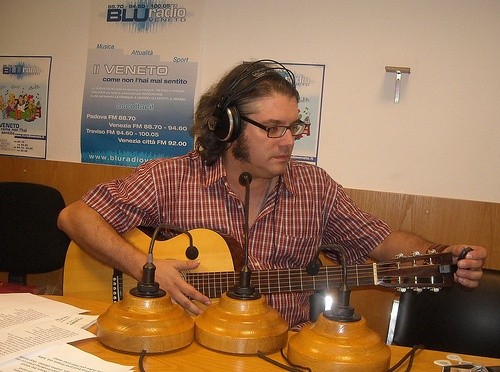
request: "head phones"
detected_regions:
[208,60,295,143]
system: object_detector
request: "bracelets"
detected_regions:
[424,244,450,266]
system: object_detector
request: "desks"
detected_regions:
[40,294,500,372]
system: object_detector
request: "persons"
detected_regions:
[57,59,486,333]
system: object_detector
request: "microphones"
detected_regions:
[306,245,354,317]
[137,224,199,292]
[234,172,255,294]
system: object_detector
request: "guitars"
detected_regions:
[62,221,473,303]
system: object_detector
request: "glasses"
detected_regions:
[241,115,306,138]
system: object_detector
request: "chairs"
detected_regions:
[0,181,70,287]
[392,270,500,359]
